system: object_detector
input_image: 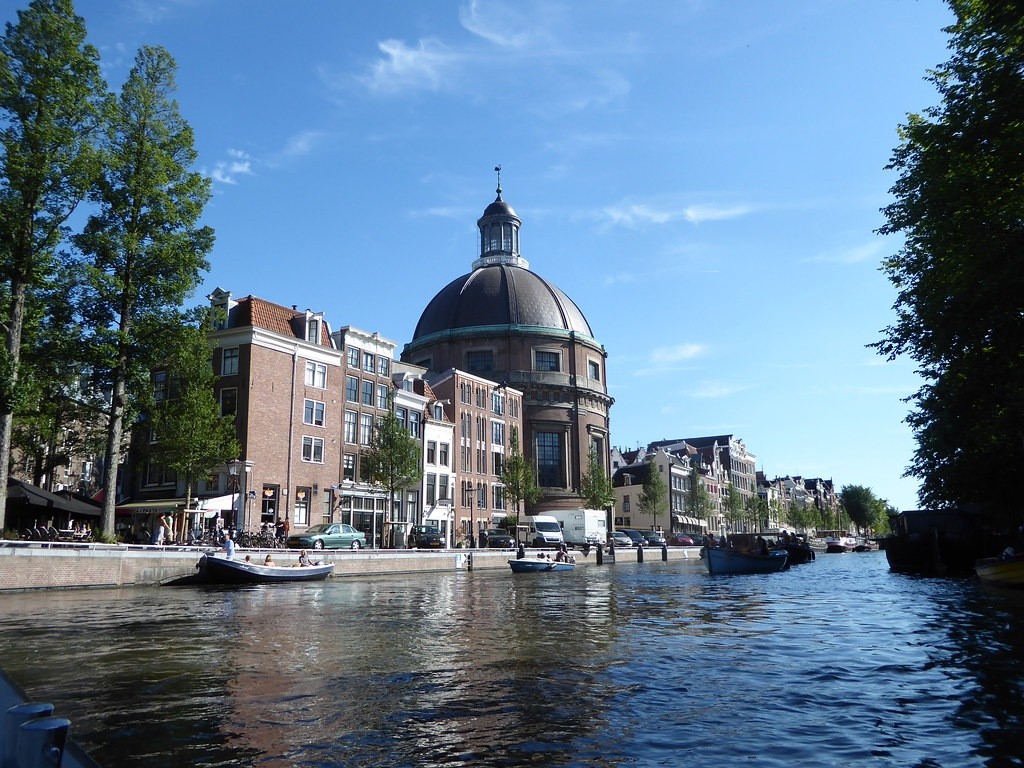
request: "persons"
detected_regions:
[555,546,571,562]
[779,531,796,543]
[68,519,86,538]
[243,555,253,565]
[261,520,271,534]
[152,511,173,545]
[998,543,1019,561]
[264,554,275,567]
[753,536,775,555]
[223,534,235,560]
[299,550,314,567]
[275,517,289,542]
[699,533,736,560]
[517,543,525,559]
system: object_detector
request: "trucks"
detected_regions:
[538,508,608,550]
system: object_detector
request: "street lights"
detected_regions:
[223,457,244,547]
[465,485,479,548]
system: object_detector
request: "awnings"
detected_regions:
[675,515,707,526]
[114,500,196,513]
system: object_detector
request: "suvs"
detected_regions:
[408,524,446,550]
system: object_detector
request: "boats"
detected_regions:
[806,529,885,553]
[973,552,1024,585]
[195,549,337,584]
[701,531,790,576]
[767,533,815,565]
[507,553,577,573]
[869,505,991,577]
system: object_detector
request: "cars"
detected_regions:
[607,529,721,549]
[479,527,516,551]
[286,522,367,552]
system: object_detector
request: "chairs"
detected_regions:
[26,527,91,549]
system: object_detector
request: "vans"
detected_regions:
[516,515,565,550]
